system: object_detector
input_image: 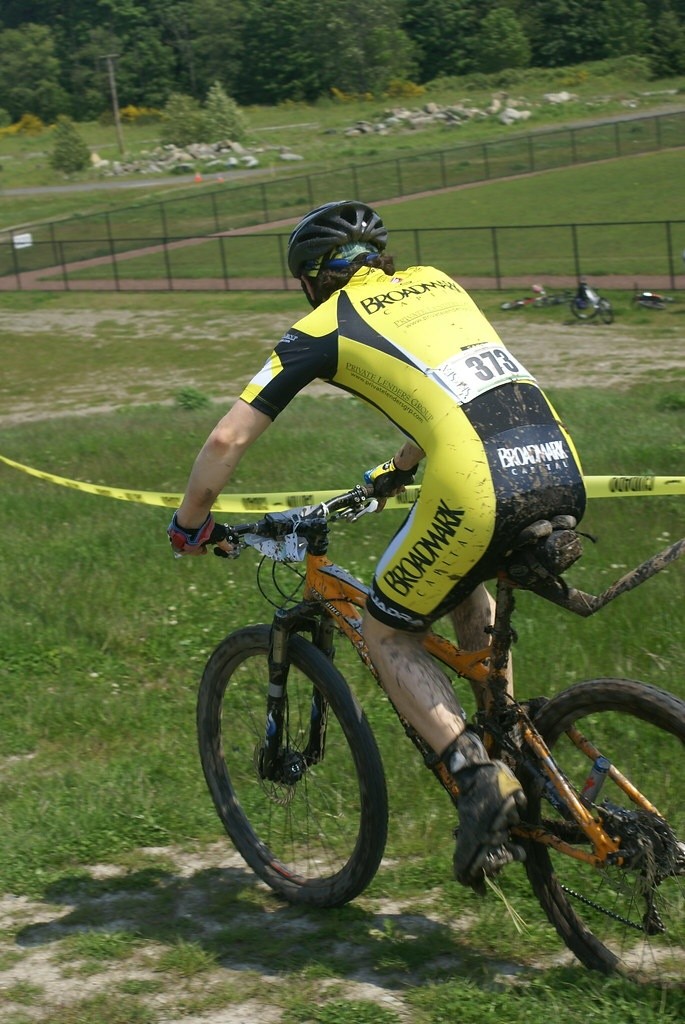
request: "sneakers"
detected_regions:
[454,762,525,886]
[481,720,521,770]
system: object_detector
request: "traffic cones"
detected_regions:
[193,168,203,184]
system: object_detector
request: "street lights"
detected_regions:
[98,53,127,155]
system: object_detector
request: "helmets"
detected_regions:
[287,200,389,281]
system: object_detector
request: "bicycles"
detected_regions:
[498,283,566,312]
[196,461,685,998]
[629,280,676,311]
[568,278,615,324]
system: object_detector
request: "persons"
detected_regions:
[168,200,590,888]
